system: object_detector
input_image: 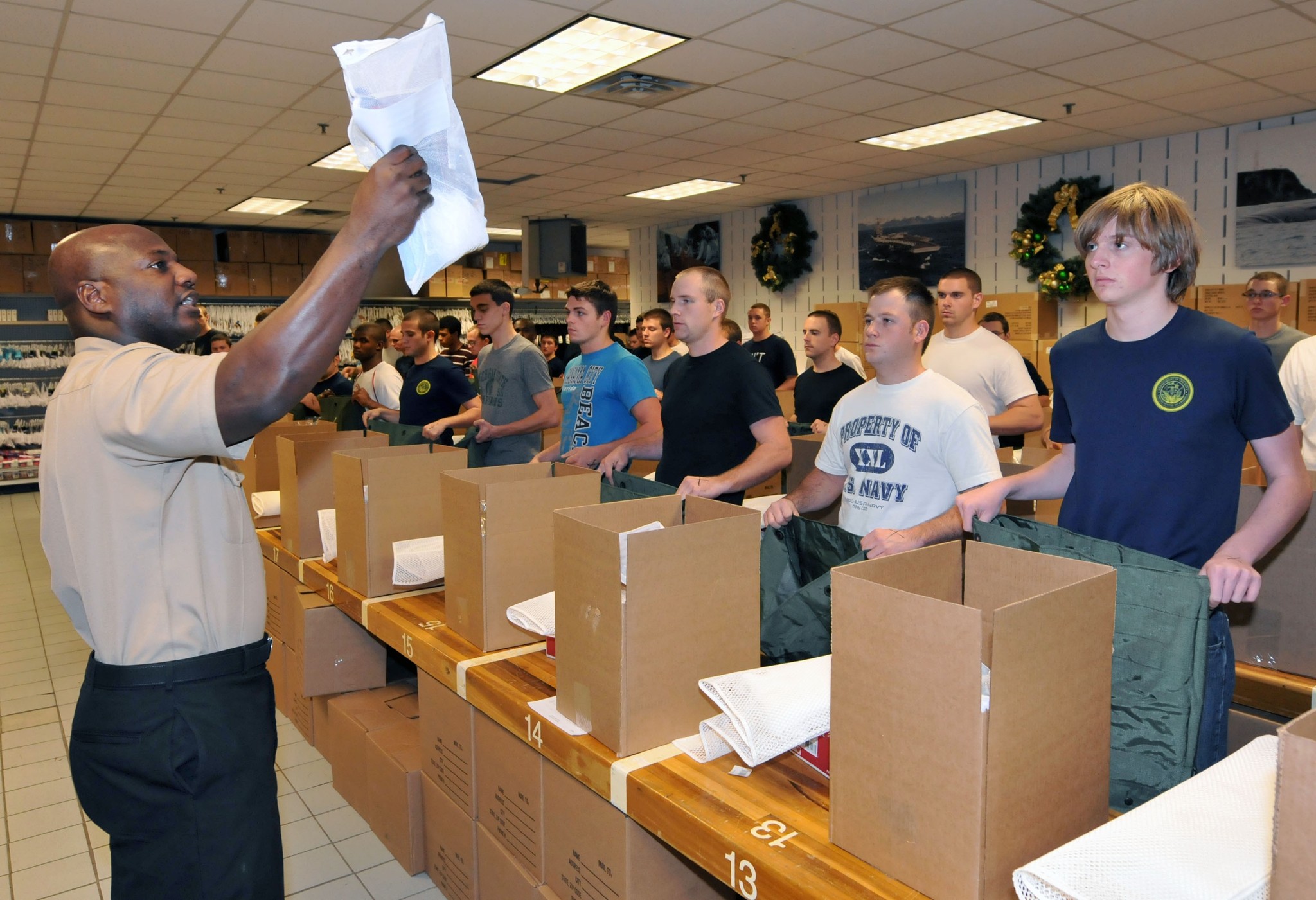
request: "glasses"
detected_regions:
[1242,290,1285,300]
[992,331,1005,337]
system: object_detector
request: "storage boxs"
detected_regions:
[0,278,1316,900]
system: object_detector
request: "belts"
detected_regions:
[88,632,273,687]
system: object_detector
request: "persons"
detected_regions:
[719,318,742,345]
[512,318,538,344]
[362,309,480,441]
[255,308,353,420]
[466,324,492,379]
[742,303,798,391]
[954,183,1316,774]
[597,266,791,498]
[39,144,433,900]
[375,318,415,380]
[629,308,689,401]
[541,335,565,378]
[354,322,403,421]
[791,310,868,436]
[527,279,662,469]
[1241,271,1316,474]
[467,279,560,469]
[763,276,1006,559]
[920,267,1051,464]
[195,305,232,355]
[438,315,474,375]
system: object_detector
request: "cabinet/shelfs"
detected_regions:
[0,293,109,490]
